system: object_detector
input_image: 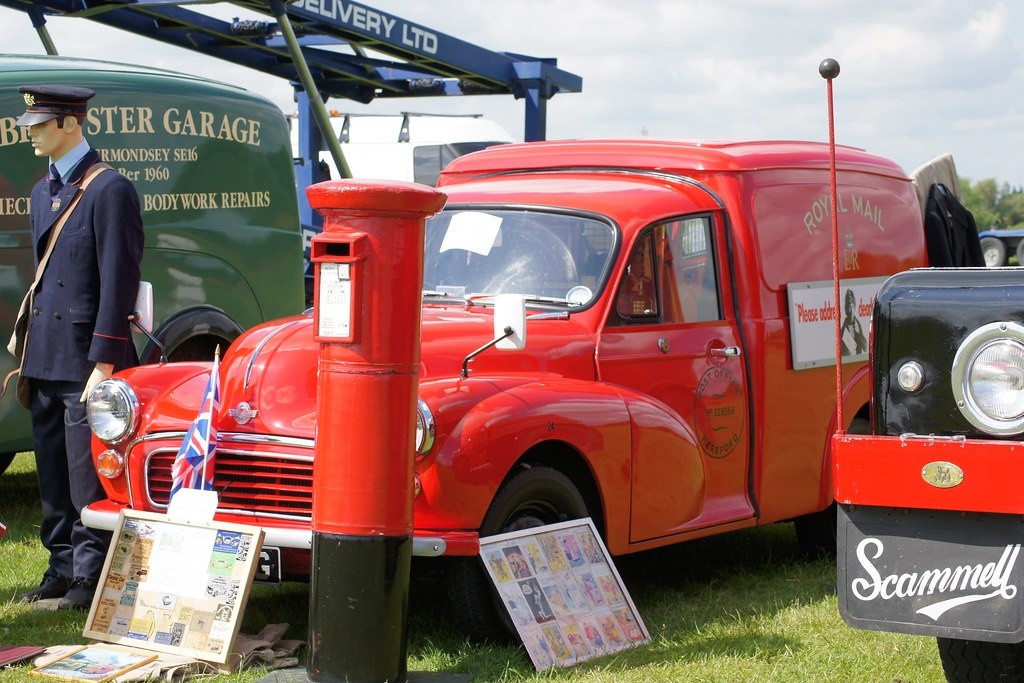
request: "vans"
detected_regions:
[283,107,528,273]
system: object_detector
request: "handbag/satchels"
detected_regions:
[0,285,35,409]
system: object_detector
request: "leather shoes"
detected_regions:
[20,574,68,603]
[57,577,97,613]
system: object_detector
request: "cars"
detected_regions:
[73,137,933,644]
[0,50,309,480]
[816,58,1024,683]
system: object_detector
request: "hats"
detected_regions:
[16,85,95,126]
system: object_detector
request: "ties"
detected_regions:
[48,164,63,197]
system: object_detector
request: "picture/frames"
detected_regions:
[82,508,267,663]
[476,517,653,674]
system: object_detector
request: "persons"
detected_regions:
[13,85,145,608]
[841,289,867,355]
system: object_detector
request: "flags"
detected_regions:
[168,355,227,513]
